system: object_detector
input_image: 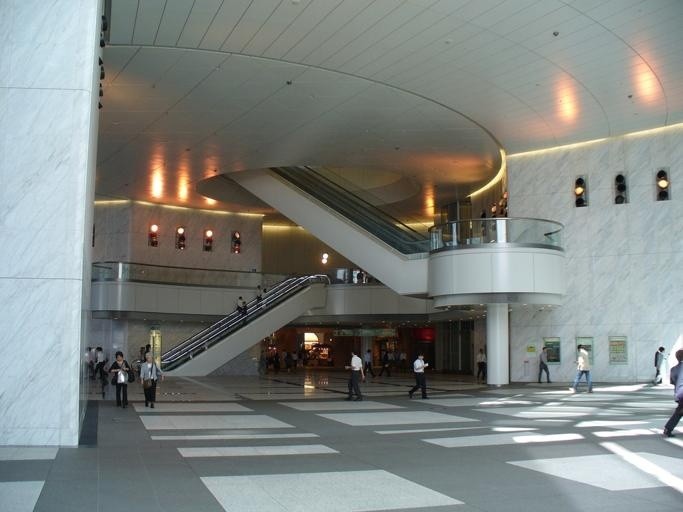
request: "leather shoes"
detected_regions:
[354,398,361,400]
[422,397,429,399]
[663,428,674,437]
[409,391,411,398]
[344,398,352,400]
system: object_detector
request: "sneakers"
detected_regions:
[145,402,148,407]
[569,388,576,392]
[151,404,154,408]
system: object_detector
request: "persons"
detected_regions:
[261,344,309,375]
[238,296,244,312]
[346,350,365,400]
[475,348,488,382]
[257,284,264,302]
[569,345,594,394]
[261,287,268,305]
[357,268,364,284]
[86,344,164,409]
[241,301,250,322]
[653,345,683,436]
[538,346,551,383]
[408,354,430,400]
[363,347,396,377]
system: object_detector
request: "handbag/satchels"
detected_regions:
[128,370,135,382]
[111,372,118,384]
[143,378,152,389]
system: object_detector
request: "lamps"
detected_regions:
[615,175,626,204]
[203,229,212,253]
[175,227,185,249]
[575,175,587,207]
[480,190,509,235]
[655,167,670,200]
[148,225,158,247]
[230,230,240,254]
